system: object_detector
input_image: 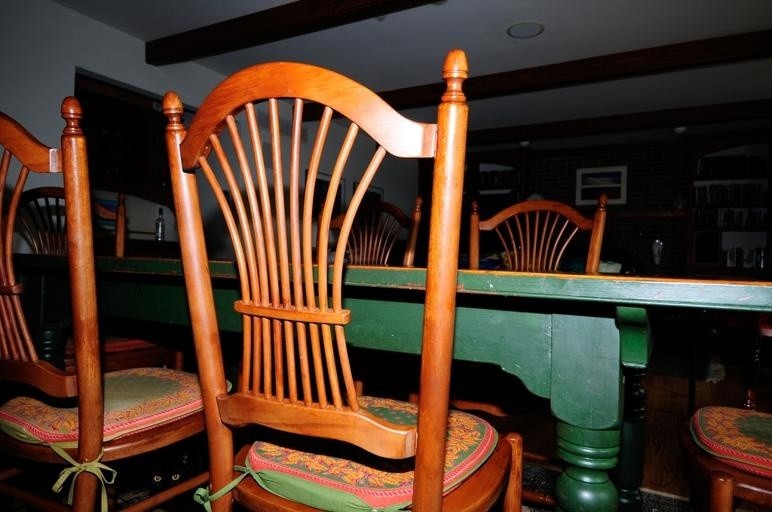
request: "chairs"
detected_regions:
[3,186,129,258]
[1,96,233,512]
[682,404,772,512]
[160,54,529,512]
[318,196,425,267]
[468,194,610,276]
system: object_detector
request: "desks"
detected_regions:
[15,246,772,507]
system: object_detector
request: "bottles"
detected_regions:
[155,208,165,241]
